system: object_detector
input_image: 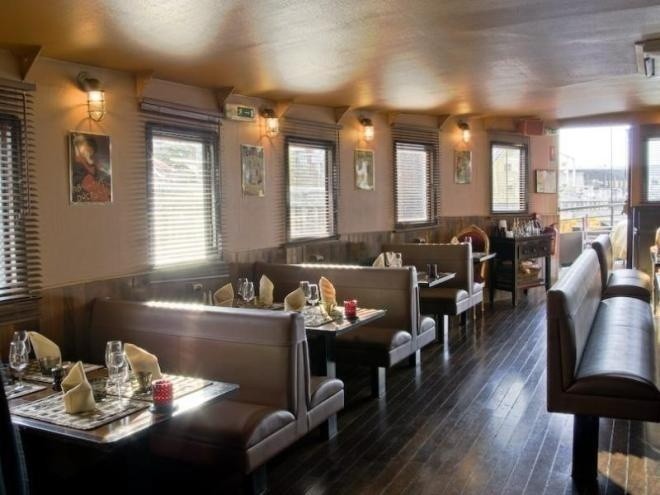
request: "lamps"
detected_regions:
[261,108,280,139]
[459,121,470,144]
[359,118,374,141]
[77,69,104,121]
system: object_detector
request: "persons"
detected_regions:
[70,133,111,203]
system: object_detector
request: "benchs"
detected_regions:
[545,234,660,495]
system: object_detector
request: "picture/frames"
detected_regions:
[535,168,557,194]
[454,149,472,184]
[241,143,265,197]
[353,149,375,192]
[68,129,114,205]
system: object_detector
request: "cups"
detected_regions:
[151,379,173,409]
[39,355,61,375]
[343,300,358,320]
[511,216,541,237]
[427,263,437,280]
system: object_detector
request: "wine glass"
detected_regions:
[103,338,128,410]
[8,330,32,393]
[236,277,255,306]
[299,281,320,307]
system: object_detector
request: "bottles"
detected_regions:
[494,226,505,237]
[50,361,72,391]
